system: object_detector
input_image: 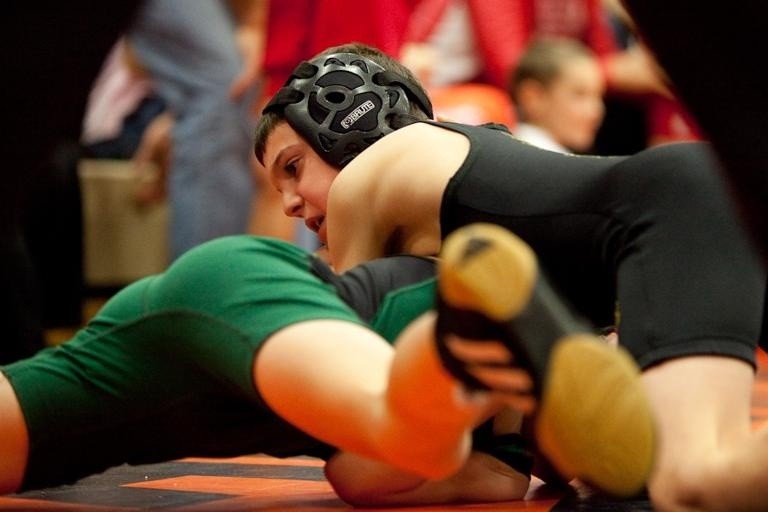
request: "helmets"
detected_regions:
[262,52,434,171]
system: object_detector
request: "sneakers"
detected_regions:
[433,222,658,495]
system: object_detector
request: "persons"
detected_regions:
[0,0,703,366]
[0,223,657,511]
[254,44,767,509]
